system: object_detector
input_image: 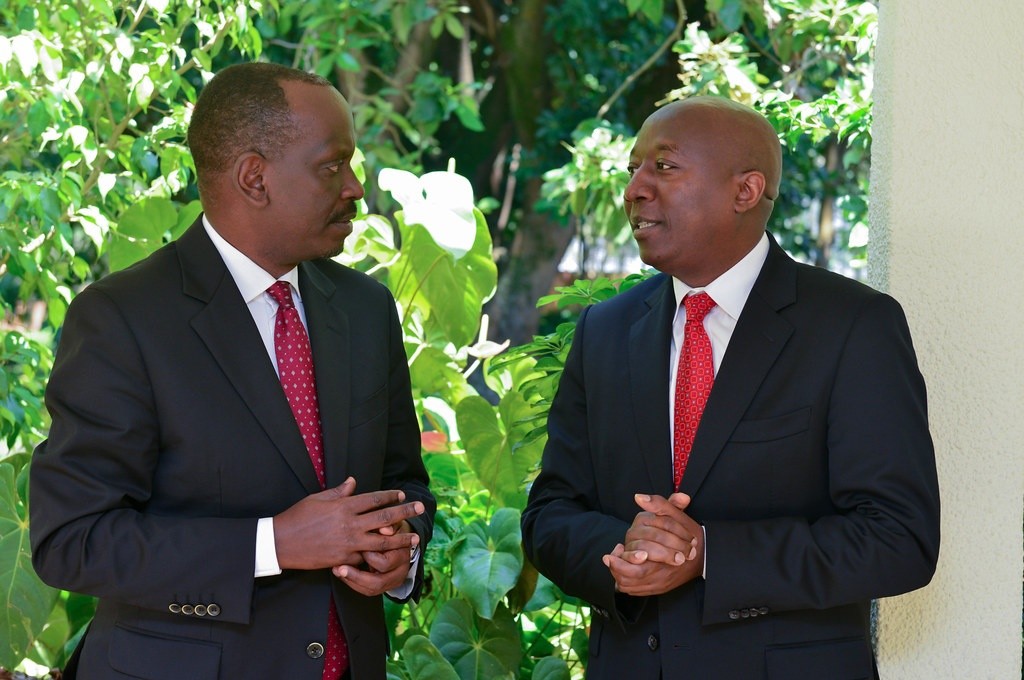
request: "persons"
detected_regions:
[516,93,946,680]
[26,63,438,680]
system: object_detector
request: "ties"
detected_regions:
[673,291,720,487]
[271,281,346,680]
